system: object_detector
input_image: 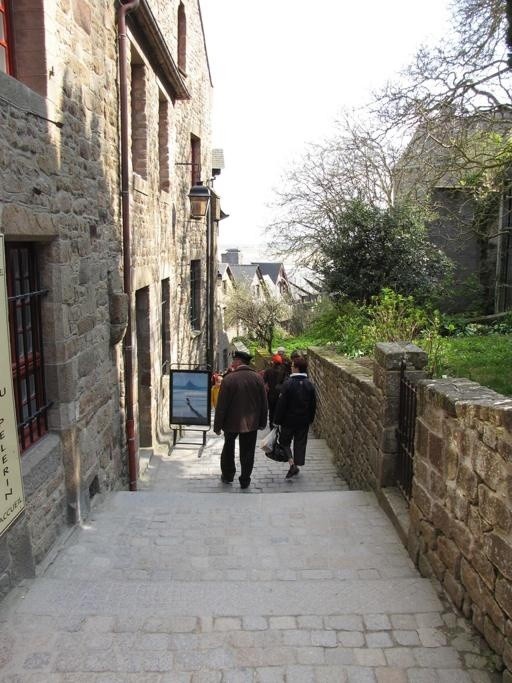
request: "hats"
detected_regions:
[232,351,252,364]
[272,355,282,365]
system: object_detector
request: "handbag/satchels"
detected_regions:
[265,429,288,462]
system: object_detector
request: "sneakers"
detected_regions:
[286,467,299,478]
[238,476,251,489]
[221,475,233,483]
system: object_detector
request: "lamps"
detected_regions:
[175,162,212,219]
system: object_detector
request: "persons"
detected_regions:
[272,357,317,479]
[213,351,268,489]
[211,346,310,433]
